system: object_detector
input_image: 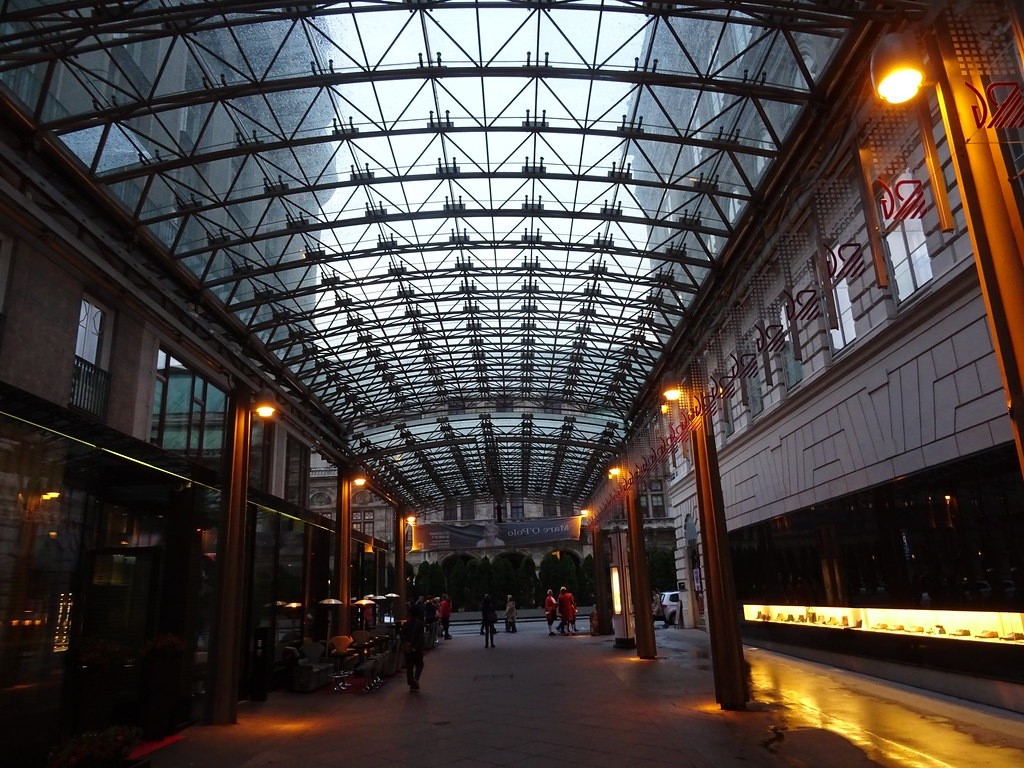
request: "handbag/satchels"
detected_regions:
[404,642,412,653]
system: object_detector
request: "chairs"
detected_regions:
[277,620,439,692]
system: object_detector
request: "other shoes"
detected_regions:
[410,686,415,692]
[415,686,419,692]
[485,645,488,648]
[491,645,495,648]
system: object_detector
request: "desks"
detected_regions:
[305,662,334,685]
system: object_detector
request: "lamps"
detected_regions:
[341,455,366,487]
[400,499,417,522]
[234,376,276,417]
[661,359,691,400]
[869,33,945,106]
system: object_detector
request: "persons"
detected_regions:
[480,594,517,649]
[476,524,506,546]
[407,594,452,648]
[652,589,659,621]
[545,589,558,636]
[400,609,424,689]
[556,587,578,636]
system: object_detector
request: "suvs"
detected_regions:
[659,591,683,626]
